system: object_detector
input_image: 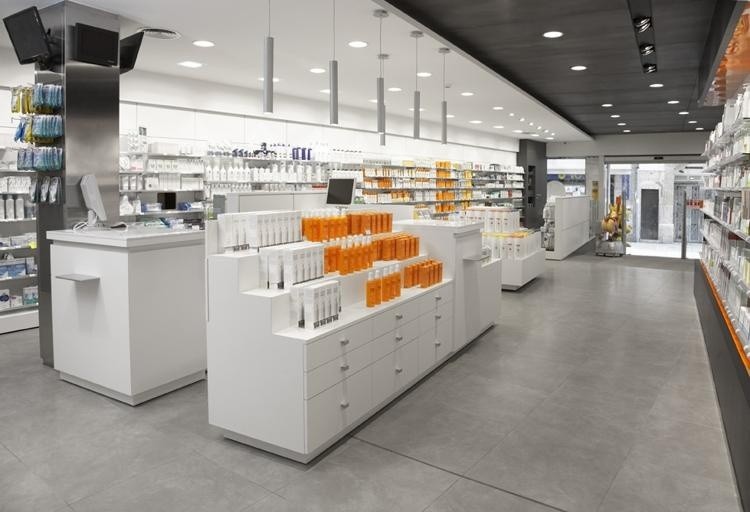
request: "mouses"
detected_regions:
[110,221,127,228]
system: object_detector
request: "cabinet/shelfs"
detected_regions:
[46,226,207,407]
[205,204,501,466]
[204,144,523,221]
[121,130,207,231]
[0,124,63,335]
[541,196,589,260]
[447,204,543,291]
[698,0,750,377]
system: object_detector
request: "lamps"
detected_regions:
[494,125,503,130]
[551,132,555,136]
[545,129,548,132]
[532,133,539,137]
[635,16,656,73]
[538,126,543,130]
[380,105,386,146]
[546,137,550,141]
[514,130,523,134]
[440,101,447,144]
[378,78,385,133]
[414,92,420,140]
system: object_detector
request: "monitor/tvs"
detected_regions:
[326,178,356,208]
[80,175,111,230]
[3,6,52,65]
[157,192,176,210]
[120,32,144,73]
[74,22,119,66]
